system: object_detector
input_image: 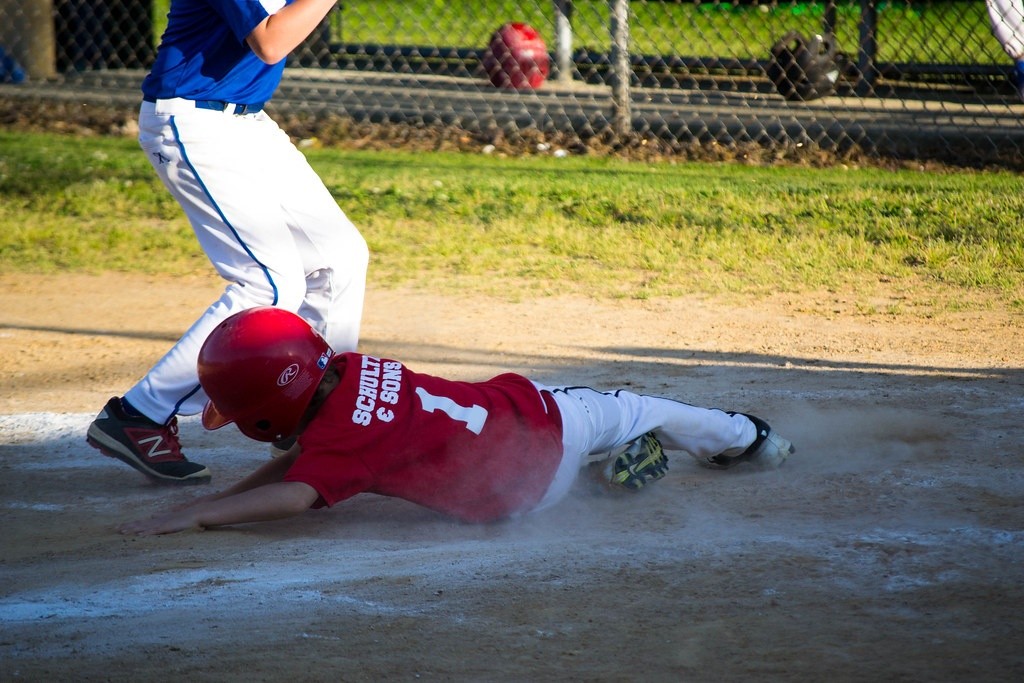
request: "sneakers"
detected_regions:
[698,410,795,473]
[595,431,669,492]
[268,437,299,459]
[87,396,211,489]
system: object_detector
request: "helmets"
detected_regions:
[197,306,336,442]
[767,30,840,101]
[482,22,548,89]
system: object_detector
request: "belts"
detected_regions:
[143,94,265,114]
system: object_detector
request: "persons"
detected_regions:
[84,0,371,487]
[984,0,1024,75]
[104,306,797,538]
